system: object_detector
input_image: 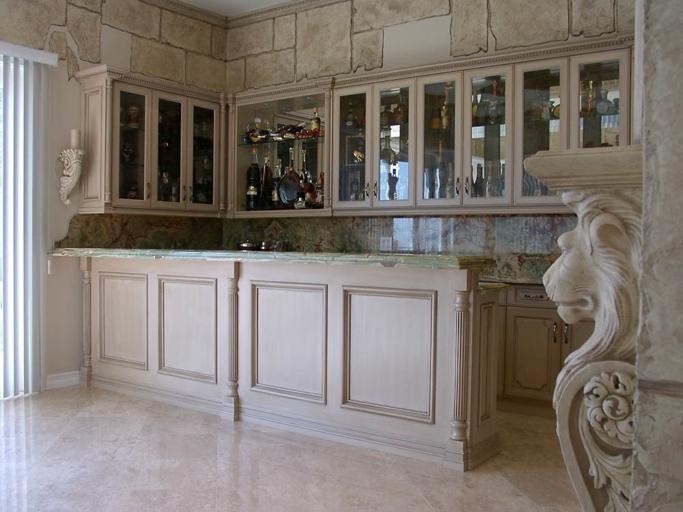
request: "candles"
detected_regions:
[70,129,81,148]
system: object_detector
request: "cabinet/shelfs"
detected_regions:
[152,82,228,217]
[500,285,602,417]
[75,65,152,216]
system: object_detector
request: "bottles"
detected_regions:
[245,148,323,209]
[309,107,320,137]
[333,80,619,202]
[120,107,211,205]
[246,118,269,144]
[238,239,270,251]
